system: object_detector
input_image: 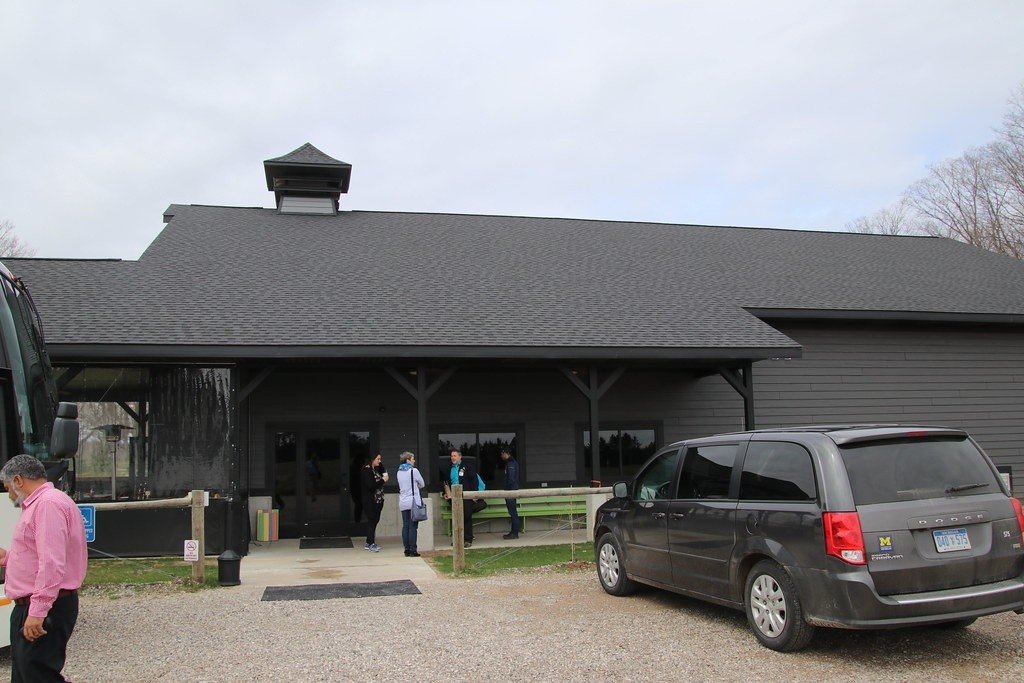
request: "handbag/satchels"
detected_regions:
[411,499,427,521]
[472,499,487,513]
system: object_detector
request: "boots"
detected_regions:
[410,546,420,557]
[404,546,410,556]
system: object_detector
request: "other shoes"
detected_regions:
[464,542,472,547]
[472,536,475,539]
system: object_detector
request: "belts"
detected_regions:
[14,589,77,605]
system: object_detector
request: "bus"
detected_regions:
[0,259,82,654]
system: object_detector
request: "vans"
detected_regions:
[594,423,1024,652]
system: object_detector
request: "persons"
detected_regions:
[444,449,480,548]
[361,452,389,553]
[306,453,319,502]
[397,451,425,557]
[0,454,88,683]
[501,445,520,540]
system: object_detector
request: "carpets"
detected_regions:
[299,537,354,549]
[260,579,423,601]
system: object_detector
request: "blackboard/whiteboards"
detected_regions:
[256,509,279,542]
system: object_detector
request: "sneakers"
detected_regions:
[365,543,382,549]
[504,532,518,539]
[369,543,379,552]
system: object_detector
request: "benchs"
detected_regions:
[439,486,591,538]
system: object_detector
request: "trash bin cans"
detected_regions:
[217,549,242,586]
